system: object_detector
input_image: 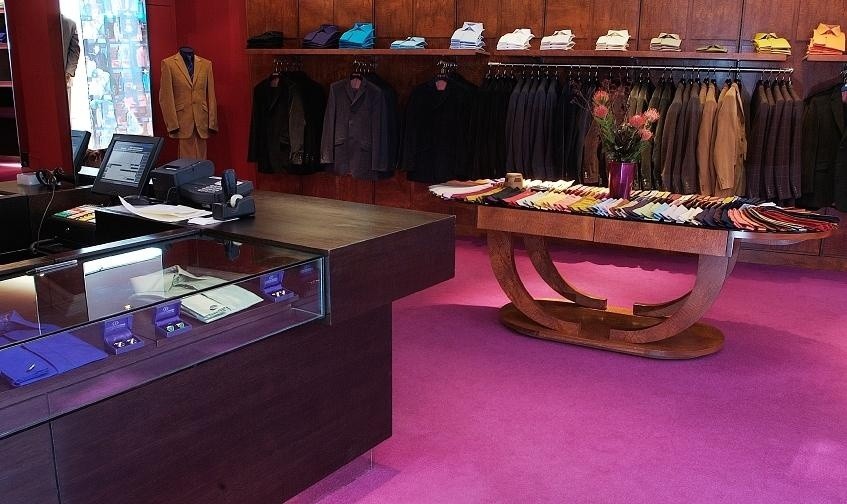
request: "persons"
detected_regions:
[59,13,81,115]
[160,47,219,160]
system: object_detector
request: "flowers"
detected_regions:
[569,80,662,163]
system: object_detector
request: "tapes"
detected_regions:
[230,195,244,208]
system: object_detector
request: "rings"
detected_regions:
[165,322,185,331]
[271,290,285,298]
[113,338,136,347]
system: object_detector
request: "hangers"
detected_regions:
[268,54,462,79]
[487,63,655,81]
[725,67,743,84]
[758,68,792,85]
[662,67,717,84]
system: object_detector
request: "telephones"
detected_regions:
[222,169,237,203]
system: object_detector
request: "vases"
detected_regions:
[607,159,635,201]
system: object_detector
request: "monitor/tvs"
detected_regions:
[71,130,91,174]
[91,133,164,197]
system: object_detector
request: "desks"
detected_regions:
[438,197,838,360]
